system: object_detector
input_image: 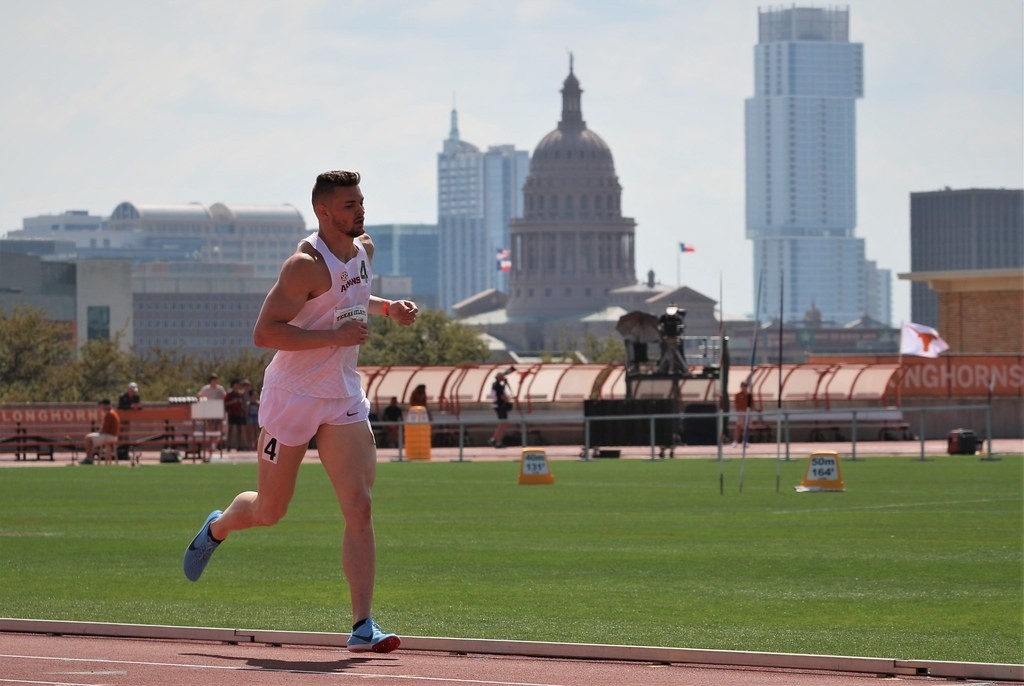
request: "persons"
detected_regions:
[729,380,755,448]
[364,383,434,450]
[626,302,691,376]
[487,372,513,449]
[116,382,142,461]
[79,397,121,465]
[196,372,261,456]
[182,169,404,655]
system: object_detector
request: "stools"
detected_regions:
[97,441,120,465]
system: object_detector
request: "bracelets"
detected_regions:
[382,299,394,320]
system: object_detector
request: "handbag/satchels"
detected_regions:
[161,449,181,462]
[507,402,513,412]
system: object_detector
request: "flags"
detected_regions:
[495,246,513,271]
[900,320,950,358]
[679,241,696,253]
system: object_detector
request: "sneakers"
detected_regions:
[346,619,401,652]
[184,511,226,581]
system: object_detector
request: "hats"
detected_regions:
[99,399,112,407]
[242,380,250,386]
[129,383,138,392]
[741,383,748,386]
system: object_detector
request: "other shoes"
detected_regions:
[496,444,506,448]
[745,443,748,447]
[731,442,738,447]
[81,459,92,464]
[490,440,496,447]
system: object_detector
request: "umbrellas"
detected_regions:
[617,311,661,345]
[579,307,631,337]
